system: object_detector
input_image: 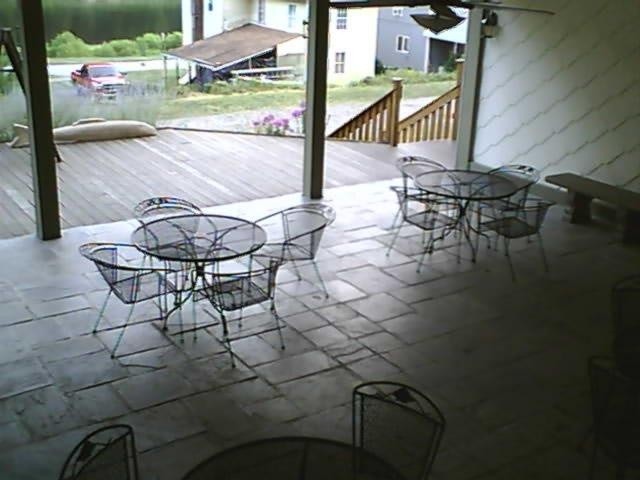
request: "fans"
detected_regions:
[329,0,554,17]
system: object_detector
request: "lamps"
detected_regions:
[479,13,503,40]
[410,13,464,37]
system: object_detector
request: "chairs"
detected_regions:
[57,423,139,479]
[589,286,640,479]
[352,381,448,479]
[385,156,557,284]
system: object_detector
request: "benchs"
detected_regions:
[545,173,640,243]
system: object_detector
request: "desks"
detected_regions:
[177,435,407,480]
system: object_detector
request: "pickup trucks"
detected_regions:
[72,63,131,100]
